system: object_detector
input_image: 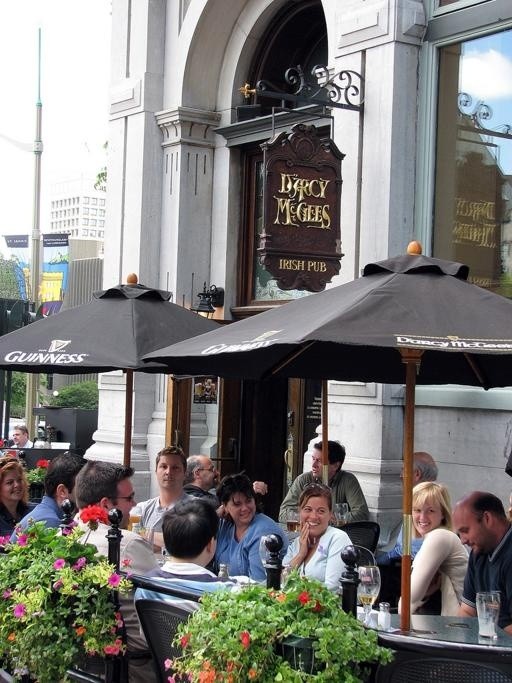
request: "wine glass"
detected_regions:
[357,565,382,628]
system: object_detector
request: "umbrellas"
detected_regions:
[0,272,225,470]
[136,241,512,633]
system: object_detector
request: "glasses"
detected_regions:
[220,474,251,485]
[112,492,136,503]
[302,481,334,493]
[0,458,18,468]
[198,466,215,473]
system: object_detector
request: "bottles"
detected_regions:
[378,600,392,631]
[217,563,230,579]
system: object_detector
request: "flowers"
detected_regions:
[163,565,395,683]
[0,503,134,683]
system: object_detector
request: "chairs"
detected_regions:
[133,598,197,682]
[351,543,378,609]
[375,651,512,683]
[338,521,380,565]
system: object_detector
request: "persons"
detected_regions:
[373,451,438,608]
[397,482,470,618]
[280,482,358,603]
[453,490,512,637]
[2,425,288,680]
[278,439,370,529]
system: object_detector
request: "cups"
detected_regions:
[286,507,300,533]
[129,505,152,543]
[475,591,502,639]
[334,502,350,527]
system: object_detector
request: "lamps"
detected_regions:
[189,281,224,319]
